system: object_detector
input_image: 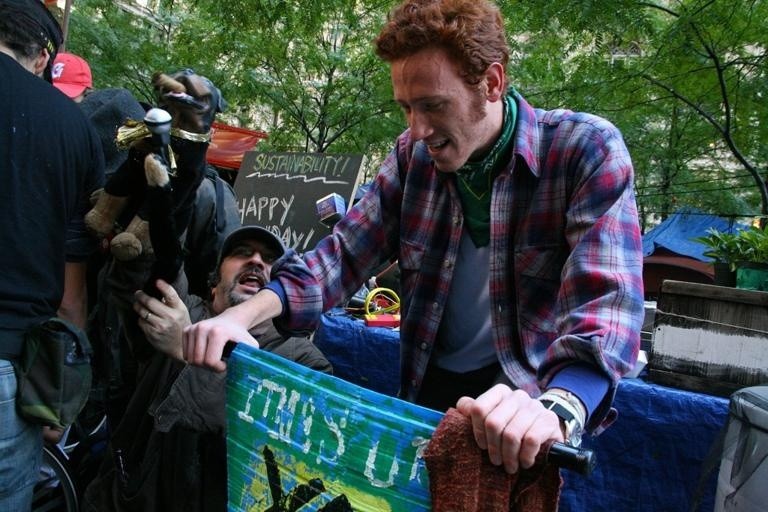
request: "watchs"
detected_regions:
[537,400,583,450]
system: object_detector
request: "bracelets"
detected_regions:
[542,392,585,425]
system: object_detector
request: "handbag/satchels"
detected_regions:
[13,314,97,430]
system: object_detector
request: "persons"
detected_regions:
[181,0,647,477]
[0,0,333,512]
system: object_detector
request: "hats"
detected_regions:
[212,225,288,272]
[0,0,65,86]
[49,52,92,99]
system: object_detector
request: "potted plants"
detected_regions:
[727,226,768,291]
[688,227,741,287]
